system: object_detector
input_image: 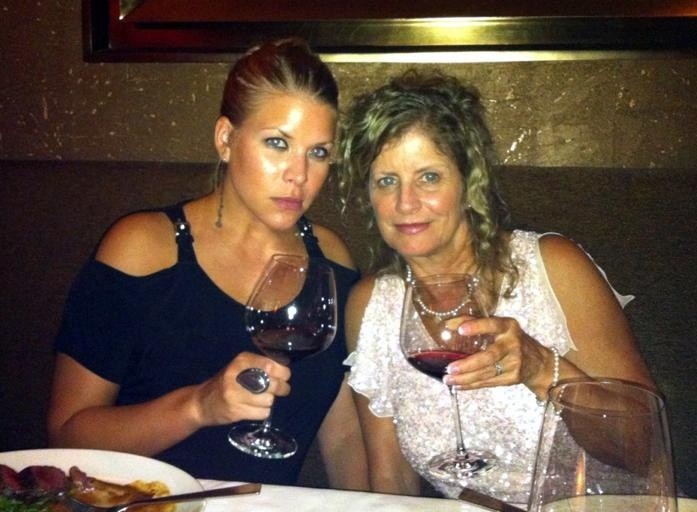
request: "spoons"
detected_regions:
[61,475,261,511]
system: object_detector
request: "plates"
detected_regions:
[4,447,209,510]
[526,495,696,511]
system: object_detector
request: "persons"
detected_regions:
[327,66,682,512]
[43,34,372,492]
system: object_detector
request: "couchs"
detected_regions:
[0,163,697,512]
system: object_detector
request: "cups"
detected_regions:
[527,375,677,510]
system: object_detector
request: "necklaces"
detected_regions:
[400,257,485,320]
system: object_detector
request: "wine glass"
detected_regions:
[400,273,504,481]
[227,252,339,461]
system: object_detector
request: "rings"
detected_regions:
[492,359,503,376]
[235,365,270,393]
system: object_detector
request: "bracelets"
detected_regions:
[534,342,560,407]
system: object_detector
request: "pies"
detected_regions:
[49,476,176,511]
